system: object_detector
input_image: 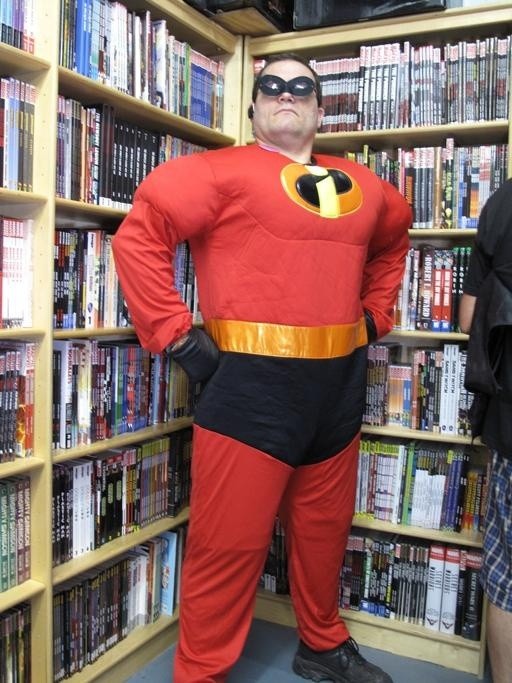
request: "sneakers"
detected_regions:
[292,636,394,682]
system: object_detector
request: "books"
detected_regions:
[1,426,194,594]
[0,0,225,132]
[353,440,491,534]
[362,343,474,439]
[256,516,288,594]
[337,533,486,642]
[254,36,510,133]
[339,137,509,230]
[0,519,189,683]
[0,76,209,213]
[1,338,207,465]
[0,215,205,330]
[395,245,473,331]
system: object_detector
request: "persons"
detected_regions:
[459,179,512,683]
[113,53,414,683]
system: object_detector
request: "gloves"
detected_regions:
[165,323,222,406]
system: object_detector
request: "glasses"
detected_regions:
[253,73,320,102]
